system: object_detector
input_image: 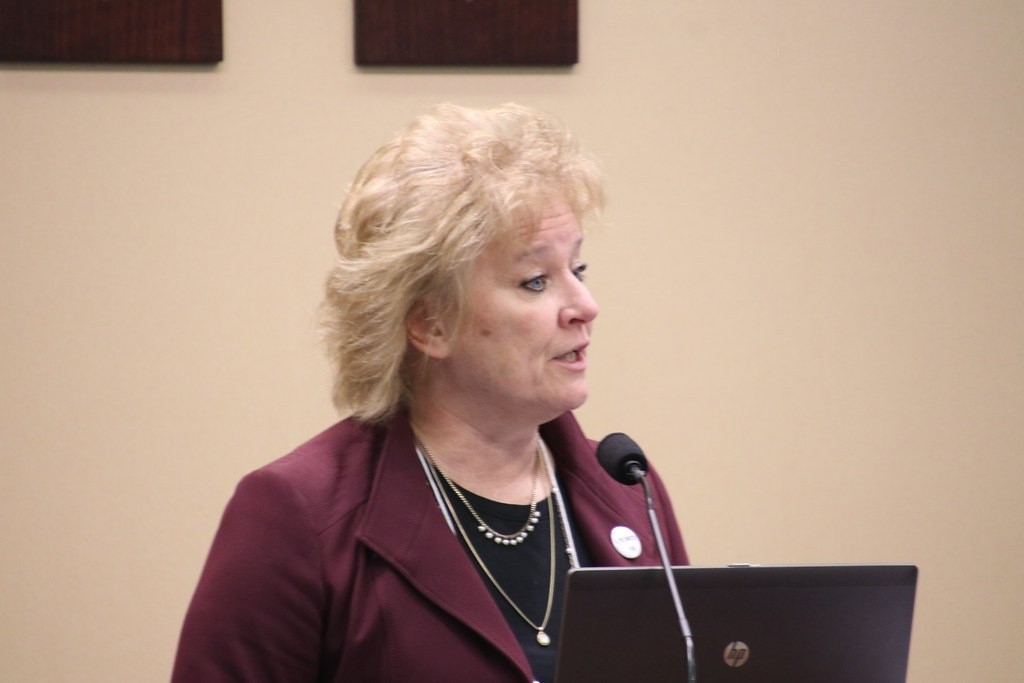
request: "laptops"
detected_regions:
[556,564,919,683]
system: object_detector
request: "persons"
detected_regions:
[171,104,690,683]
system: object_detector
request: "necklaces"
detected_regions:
[407,418,555,646]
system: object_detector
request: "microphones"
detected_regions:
[596,431,698,683]
